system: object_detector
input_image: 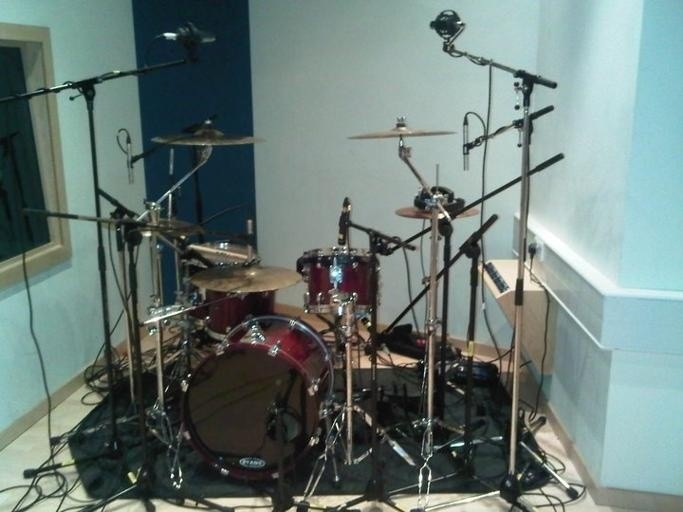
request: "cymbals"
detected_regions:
[187,239,258,298]
[191,263,300,295]
[348,127,456,140]
[395,206,478,220]
[148,128,257,147]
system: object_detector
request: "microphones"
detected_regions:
[430,20,463,28]
[156,32,216,43]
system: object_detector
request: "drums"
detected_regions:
[204,288,275,342]
[297,247,381,316]
[180,314,334,484]
[187,285,210,320]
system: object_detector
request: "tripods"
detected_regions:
[0,41,579,512]
